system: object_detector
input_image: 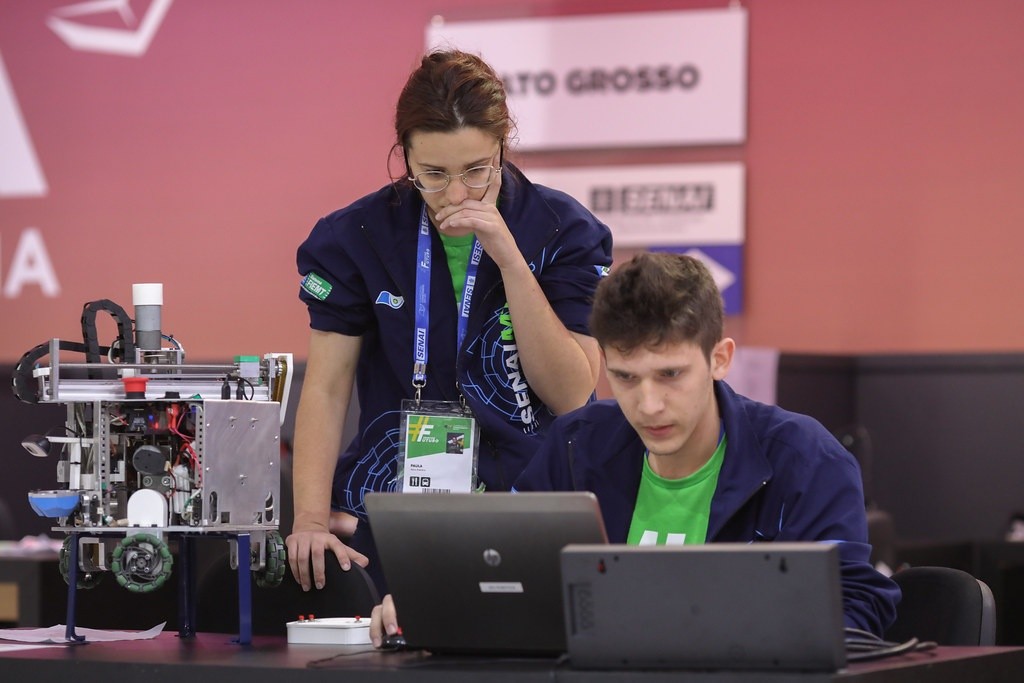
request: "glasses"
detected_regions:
[401,138,506,194]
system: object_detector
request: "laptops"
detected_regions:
[363,491,612,656]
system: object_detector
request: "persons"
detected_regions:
[286,43,612,591]
[370,251,903,649]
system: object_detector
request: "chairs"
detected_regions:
[884,567,997,647]
[192,549,381,637]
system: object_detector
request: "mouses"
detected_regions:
[381,626,407,649]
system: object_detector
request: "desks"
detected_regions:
[0,625,1024,683]
